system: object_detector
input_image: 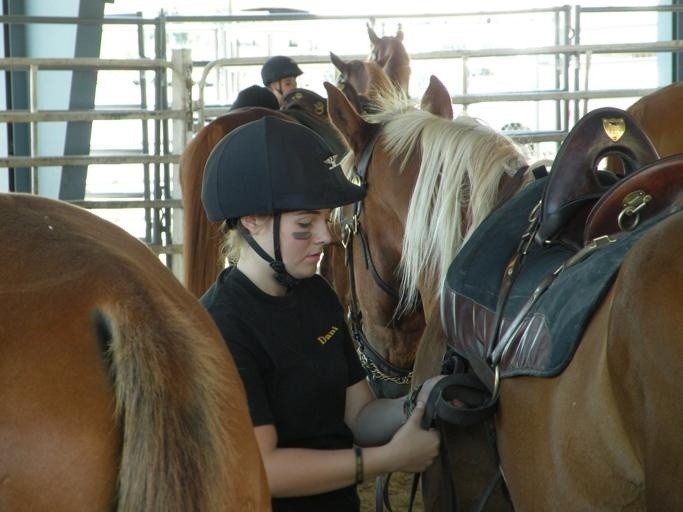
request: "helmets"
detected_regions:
[260,56,302,86]
[201,116,366,287]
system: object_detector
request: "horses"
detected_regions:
[622,82,683,157]
[365,22,411,95]
[325,76,683,509]
[0,196,270,512]
[177,52,400,294]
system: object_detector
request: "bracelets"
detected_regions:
[354,442,364,489]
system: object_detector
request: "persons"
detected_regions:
[262,54,302,99]
[184,115,466,510]
